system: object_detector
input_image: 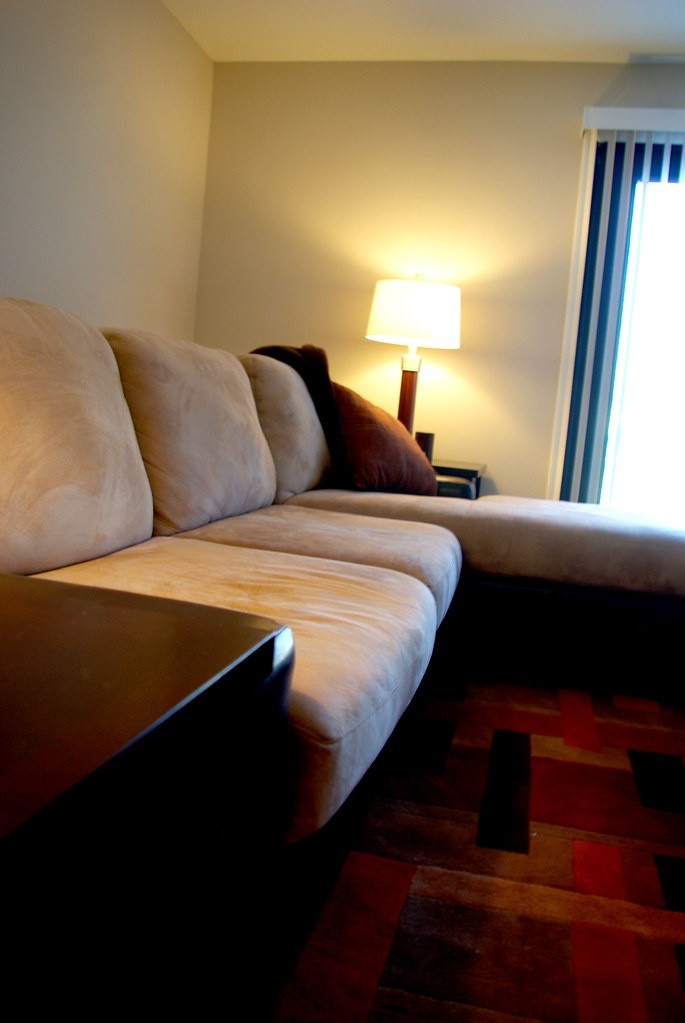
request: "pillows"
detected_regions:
[331,382,436,496]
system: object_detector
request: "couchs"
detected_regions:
[0,296,685,908]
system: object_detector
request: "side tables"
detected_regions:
[431,460,486,498]
[0,570,294,1023]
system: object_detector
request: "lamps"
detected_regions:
[365,279,461,438]
